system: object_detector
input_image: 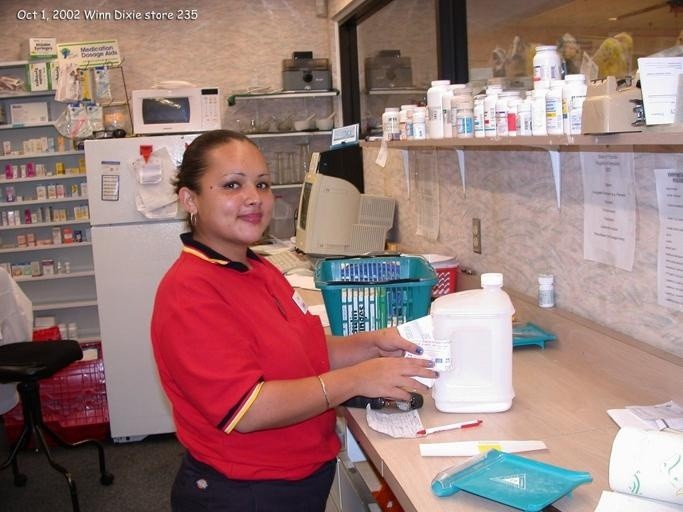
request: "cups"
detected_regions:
[273,142,313,185]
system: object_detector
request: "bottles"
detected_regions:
[531,44,563,90]
[63,228,73,243]
[381,73,587,142]
[74,231,81,243]
[537,277,555,308]
[55,258,70,275]
[79,137,220,442]
[57,322,77,342]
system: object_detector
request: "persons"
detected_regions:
[148,128,439,511]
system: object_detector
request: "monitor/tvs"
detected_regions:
[295,172,396,265]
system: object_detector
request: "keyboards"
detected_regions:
[264,250,312,276]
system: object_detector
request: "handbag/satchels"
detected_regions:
[3,341,110,451]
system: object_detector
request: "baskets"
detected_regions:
[315,255,438,336]
[432,267,457,298]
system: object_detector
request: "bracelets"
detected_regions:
[315,375,332,409]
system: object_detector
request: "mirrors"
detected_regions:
[353,0,682,134]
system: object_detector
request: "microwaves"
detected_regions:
[128,85,223,136]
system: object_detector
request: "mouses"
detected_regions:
[284,268,311,278]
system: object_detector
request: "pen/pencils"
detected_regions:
[416,419,483,434]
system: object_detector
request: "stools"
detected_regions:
[1,339,115,512]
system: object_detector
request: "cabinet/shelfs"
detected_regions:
[0,60,100,345]
[230,90,336,190]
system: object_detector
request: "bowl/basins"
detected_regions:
[294,121,315,131]
[316,120,333,131]
